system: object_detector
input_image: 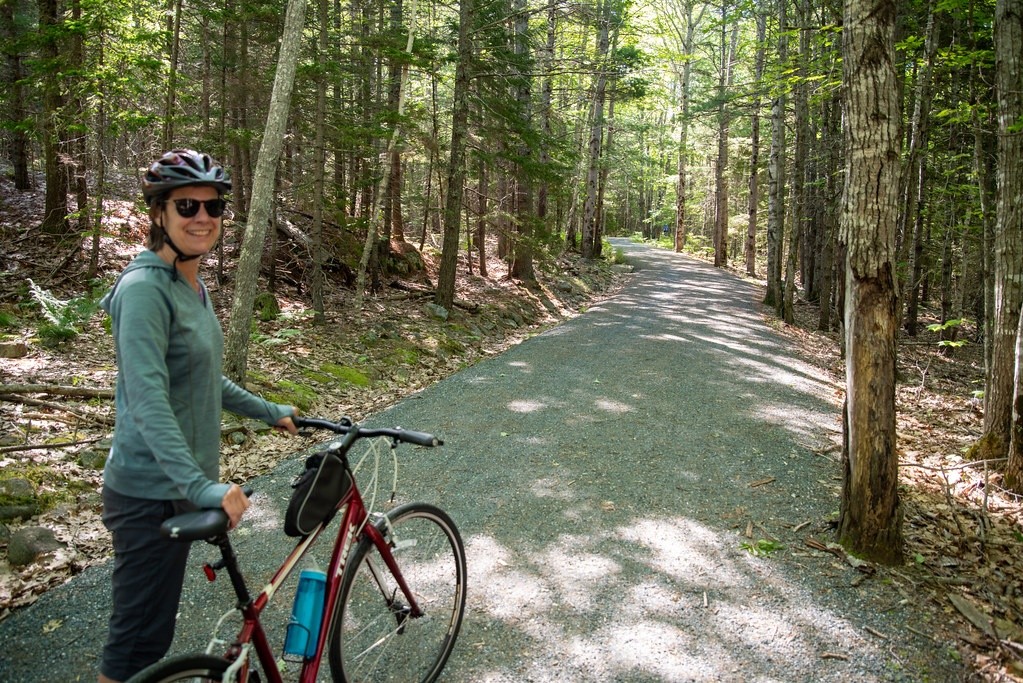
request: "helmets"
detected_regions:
[141,148,232,204]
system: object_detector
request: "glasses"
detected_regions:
[161,197,226,218]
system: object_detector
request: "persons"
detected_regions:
[98,148,301,683]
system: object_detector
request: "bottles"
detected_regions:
[286,568,327,658]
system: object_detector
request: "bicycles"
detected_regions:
[122,413,468,683]
